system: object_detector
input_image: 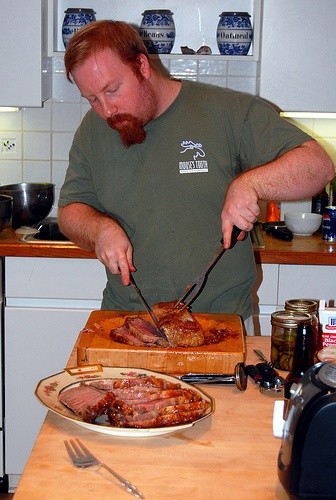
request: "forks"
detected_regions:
[171,222,246,315]
[64,439,146,500]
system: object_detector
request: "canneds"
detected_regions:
[270,300,318,371]
[322,205,336,242]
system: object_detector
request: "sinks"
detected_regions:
[251,224,266,248]
[19,216,74,245]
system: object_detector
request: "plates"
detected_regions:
[34,366,216,437]
[262,221,287,231]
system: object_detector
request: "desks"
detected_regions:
[13,336,289,500]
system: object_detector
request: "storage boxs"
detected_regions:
[319,303,336,348]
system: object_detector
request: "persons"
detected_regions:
[57,20,335,337]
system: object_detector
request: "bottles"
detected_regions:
[216,12,253,55]
[138,9,176,54]
[61,7,96,50]
[310,186,329,234]
[265,200,281,223]
[283,299,336,367]
[271,311,313,383]
[328,176,336,206]
[277,347,336,500]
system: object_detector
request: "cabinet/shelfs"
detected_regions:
[3,259,336,495]
[0,0,336,118]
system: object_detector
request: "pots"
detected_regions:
[0,182,56,229]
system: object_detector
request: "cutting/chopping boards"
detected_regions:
[77,311,247,376]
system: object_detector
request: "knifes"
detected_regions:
[126,267,169,343]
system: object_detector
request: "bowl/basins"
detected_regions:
[1,194,14,236]
[284,213,322,236]
